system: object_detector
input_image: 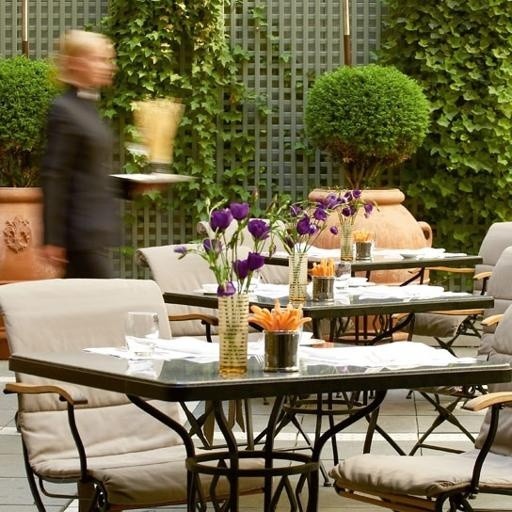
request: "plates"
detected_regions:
[401,252,418,258]
[352,282,377,287]
[300,339,326,345]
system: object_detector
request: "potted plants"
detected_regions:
[293,63,433,342]
[0,52,67,360]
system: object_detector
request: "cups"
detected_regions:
[312,276,333,303]
[124,312,160,358]
[263,330,301,372]
[334,261,351,290]
[356,241,373,262]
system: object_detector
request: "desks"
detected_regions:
[256,248,485,340]
[6,331,506,512]
[164,281,496,489]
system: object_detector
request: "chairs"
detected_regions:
[2,277,275,511]
[139,219,288,434]
[327,305,510,512]
[399,221,512,407]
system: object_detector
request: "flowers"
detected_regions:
[174,188,382,297]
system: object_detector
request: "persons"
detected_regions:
[35,29,177,279]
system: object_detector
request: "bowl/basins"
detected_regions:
[299,332,313,342]
[352,278,368,285]
